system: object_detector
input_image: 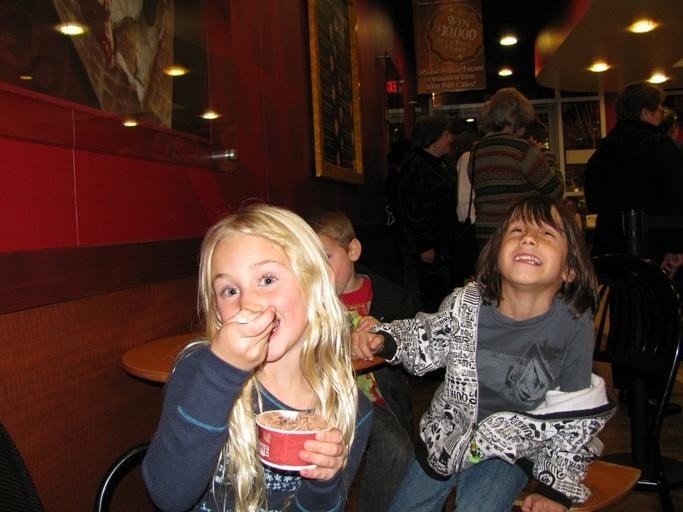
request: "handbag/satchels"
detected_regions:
[458,218,478,265]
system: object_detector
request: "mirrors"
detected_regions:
[375,48,406,182]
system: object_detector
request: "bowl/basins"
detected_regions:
[255,409,333,472]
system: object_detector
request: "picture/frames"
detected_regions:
[308,0,368,187]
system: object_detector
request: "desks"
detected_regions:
[120,332,386,388]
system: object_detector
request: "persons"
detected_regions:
[384,138,419,251]
[457,88,565,250]
[347,193,618,511]
[391,110,465,314]
[303,209,413,510]
[584,81,680,415]
[140,201,375,510]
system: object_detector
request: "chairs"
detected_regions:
[93,441,149,512]
[513,254,683,512]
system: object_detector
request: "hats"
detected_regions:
[415,114,448,148]
